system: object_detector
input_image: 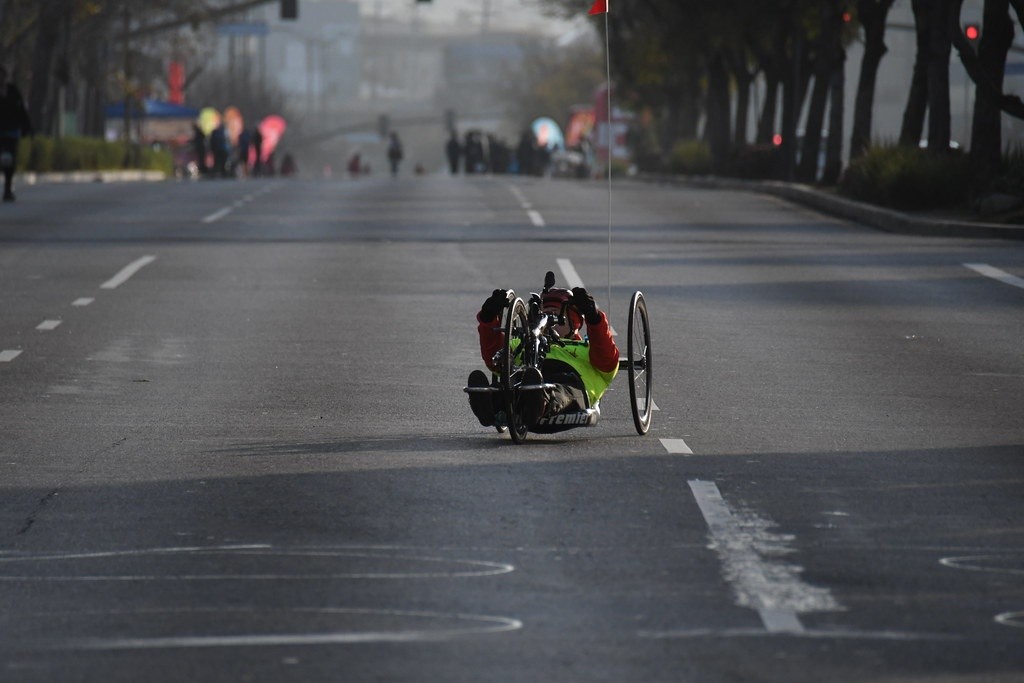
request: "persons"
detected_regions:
[351,108,593,181]
[466,287,620,426]
[0,70,33,203]
[185,120,299,181]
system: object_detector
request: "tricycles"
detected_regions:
[463,269,652,445]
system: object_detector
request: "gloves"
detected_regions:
[478,288,510,323]
[567,286,601,324]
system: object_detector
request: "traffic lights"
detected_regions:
[964,21,980,59]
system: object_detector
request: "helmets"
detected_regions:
[539,288,585,331]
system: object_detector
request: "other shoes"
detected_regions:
[518,368,550,422]
[467,370,502,427]
